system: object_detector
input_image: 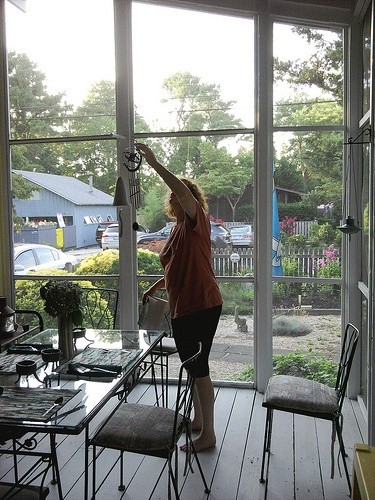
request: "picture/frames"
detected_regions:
[84,214,114,224]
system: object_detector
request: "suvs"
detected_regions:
[95,221,118,248]
[12,243,79,275]
[102,223,150,252]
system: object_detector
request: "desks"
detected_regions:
[0,327,167,500]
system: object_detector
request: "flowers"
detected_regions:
[39,279,86,327]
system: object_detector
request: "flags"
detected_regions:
[272,162,284,285]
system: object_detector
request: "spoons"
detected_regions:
[40,397,64,417]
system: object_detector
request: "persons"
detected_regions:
[134,144,223,454]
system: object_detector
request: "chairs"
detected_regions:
[124,288,178,404]
[259,322,361,485]
[79,287,119,330]
[92,342,212,500]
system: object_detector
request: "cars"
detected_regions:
[136,221,232,254]
[229,225,254,248]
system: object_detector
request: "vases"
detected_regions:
[57,313,75,358]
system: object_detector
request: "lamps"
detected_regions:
[335,125,372,242]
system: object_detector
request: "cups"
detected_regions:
[22,324,30,331]
